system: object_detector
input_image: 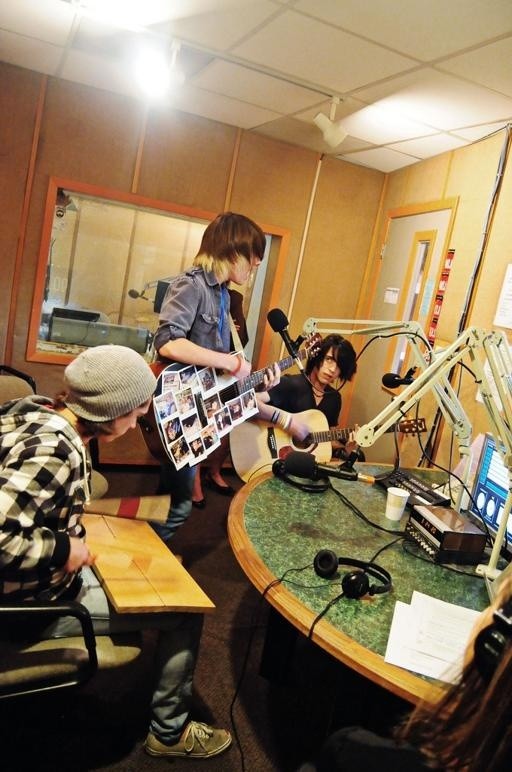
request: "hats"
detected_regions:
[63,342,158,424]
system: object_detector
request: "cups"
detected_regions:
[384,487,410,522]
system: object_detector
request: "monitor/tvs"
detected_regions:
[50,316,148,353]
[466,431,512,554]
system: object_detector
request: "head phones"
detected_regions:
[313,546,393,600]
[271,458,331,492]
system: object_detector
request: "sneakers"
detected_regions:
[145,718,233,759]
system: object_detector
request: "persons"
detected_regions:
[255,332,362,464]
[1,344,234,760]
[316,547,510,772]
[193,291,246,508]
[140,210,268,547]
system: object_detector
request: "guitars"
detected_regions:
[139,331,323,472]
[229,408,427,482]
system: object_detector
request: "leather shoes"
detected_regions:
[205,472,235,495]
[192,496,206,510]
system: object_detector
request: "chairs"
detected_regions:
[1,605,105,772]
[0,364,37,407]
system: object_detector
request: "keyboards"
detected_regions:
[374,467,451,508]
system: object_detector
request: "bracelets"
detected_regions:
[229,354,242,378]
[269,406,293,433]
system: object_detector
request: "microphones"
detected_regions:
[267,308,306,374]
[283,450,377,483]
[382,373,415,389]
[127,289,148,301]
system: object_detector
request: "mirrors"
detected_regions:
[24,176,292,378]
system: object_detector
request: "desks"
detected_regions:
[224,453,512,723]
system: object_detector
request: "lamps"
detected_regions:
[313,98,349,151]
